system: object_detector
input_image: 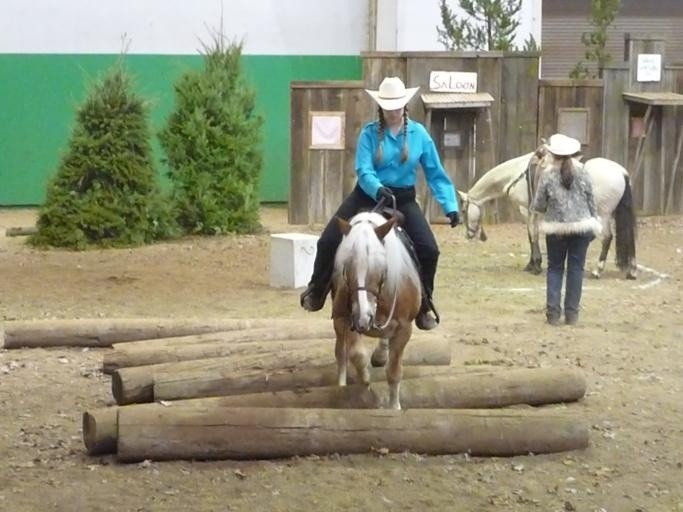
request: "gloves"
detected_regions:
[375,187,393,206]
[446,212,459,229]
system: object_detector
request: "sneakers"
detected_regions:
[416,310,438,329]
[302,280,328,310]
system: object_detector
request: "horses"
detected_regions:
[330,211,422,411]
[456,151,637,279]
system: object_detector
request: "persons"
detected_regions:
[534,134,601,325]
[302,77,460,330]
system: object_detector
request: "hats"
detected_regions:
[543,134,581,157]
[364,76,420,111]
